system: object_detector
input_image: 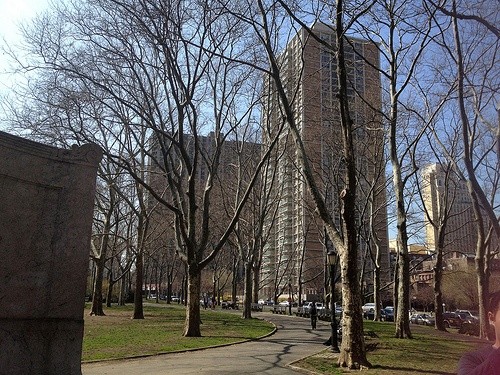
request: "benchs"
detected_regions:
[268,305,287,315]
[251,303,263,312]
[316,310,331,322]
[292,307,311,318]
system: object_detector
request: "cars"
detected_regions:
[221,299,238,310]
[251,302,263,312]
[361,302,434,326]
[147,293,157,300]
[264,299,342,322]
[441,308,480,336]
[159,294,217,307]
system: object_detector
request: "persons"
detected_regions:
[457,289,500,375]
[309,304,318,330]
